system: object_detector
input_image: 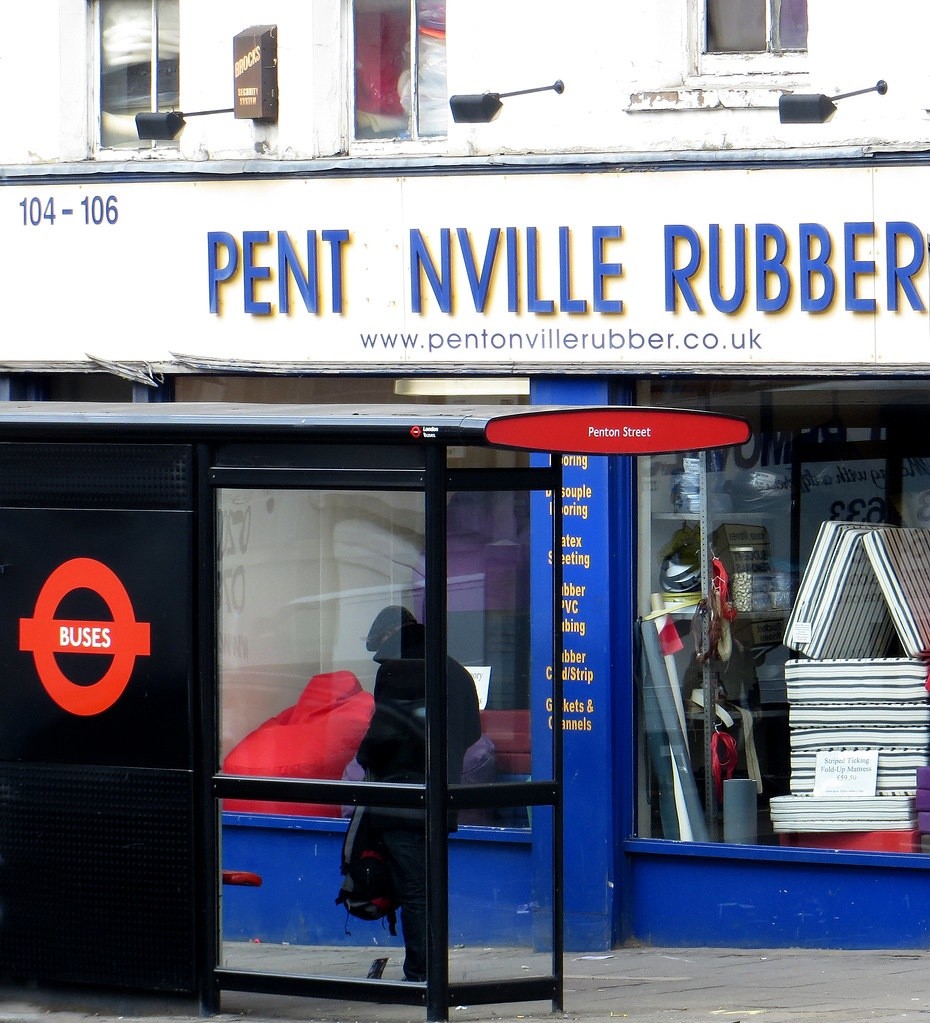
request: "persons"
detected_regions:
[356,605,482,982]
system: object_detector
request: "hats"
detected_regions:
[366,605,417,652]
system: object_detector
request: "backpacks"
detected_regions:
[335,769,407,921]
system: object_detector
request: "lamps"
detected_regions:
[449,78,564,124]
[133,107,233,140]
[778,80,887,124]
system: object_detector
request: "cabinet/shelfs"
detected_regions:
[652,509,792,721]
